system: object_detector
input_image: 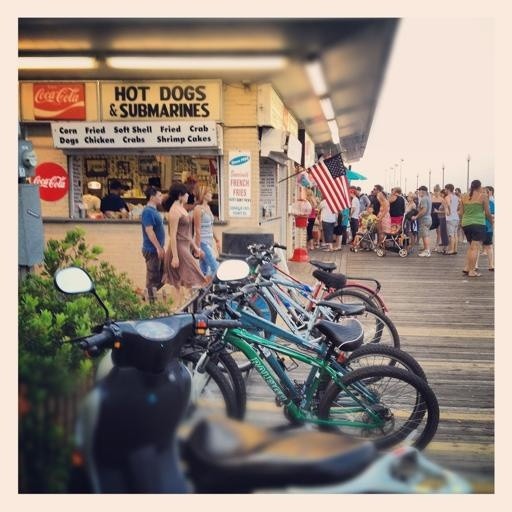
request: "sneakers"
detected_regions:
[418,251,430,257]
[433,249,458,255]
[323,247,342,251]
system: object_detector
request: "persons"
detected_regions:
[306,180,494,277]
[82,181,102,213]
[161,183,206,290]
[141,187,165,302]
[100,178,131,214]
[191,181,221,286]
[182,175,199,211]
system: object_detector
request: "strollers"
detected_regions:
[350,216,407,257]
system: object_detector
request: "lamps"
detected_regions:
[322,98,337,120]
[104,48,293,71]
[19,50,97,72]
[329,122,340,144]
[302,53,329,98]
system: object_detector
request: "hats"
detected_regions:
[88,181,101,190]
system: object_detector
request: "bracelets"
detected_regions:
[216,240,220,243]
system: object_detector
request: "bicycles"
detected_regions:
[195,241,440,450]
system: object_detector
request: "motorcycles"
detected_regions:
[45,261,473,493]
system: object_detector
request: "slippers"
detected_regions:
[460,267,494,276]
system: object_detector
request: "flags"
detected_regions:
[308,151,352,215]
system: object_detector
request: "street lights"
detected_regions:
[412,153,472,192]
[385,157,404,192]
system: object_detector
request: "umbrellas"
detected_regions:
[343,168,366,183]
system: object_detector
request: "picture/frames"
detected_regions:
[138,156,161,176]
[108,178,133,194]
[85,159,108,177]
